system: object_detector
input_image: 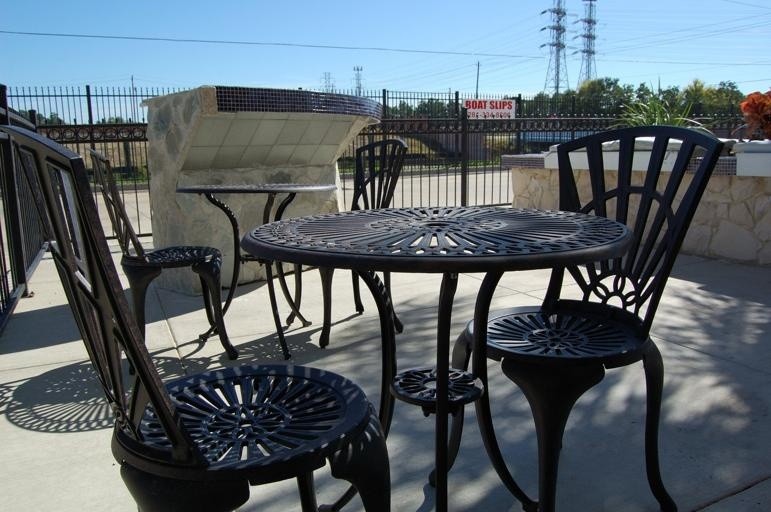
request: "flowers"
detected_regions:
[730,91,771,141]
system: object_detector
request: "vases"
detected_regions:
[732,140,770,177]
